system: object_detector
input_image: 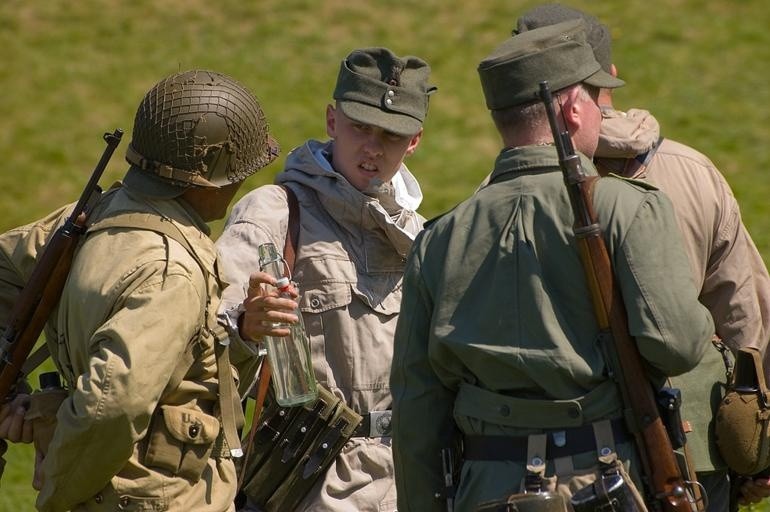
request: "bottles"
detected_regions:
[258,240,319,409]
[30,372,72,491]
[715,347,770,477]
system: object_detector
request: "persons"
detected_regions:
[470,4,770,512]
[0,67,281,512]
[389,18,715,511]
[215,47,438,511]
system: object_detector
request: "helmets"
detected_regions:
[124,68,281,189]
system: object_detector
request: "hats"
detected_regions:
[511,5,614,73]
[333,45,436,138]
[476,19,625,111]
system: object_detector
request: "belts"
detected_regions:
[353,404,395,438]
[456,420,626,463]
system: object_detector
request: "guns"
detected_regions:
[1,128,123,478]
[538,80,708,512]
